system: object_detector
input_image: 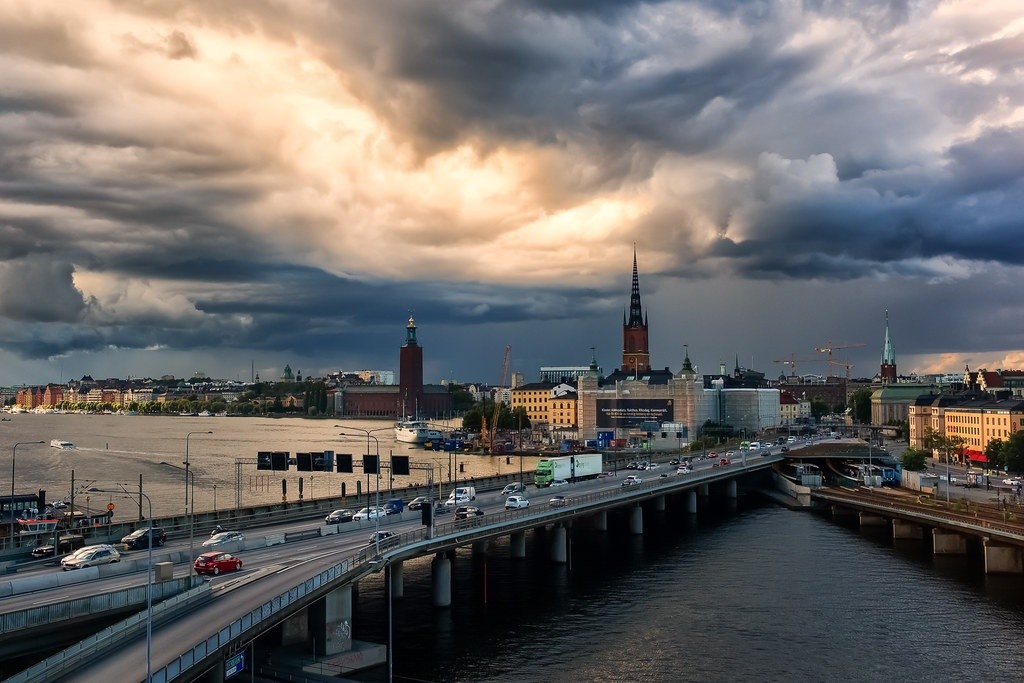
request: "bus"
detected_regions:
[859,464,896,485]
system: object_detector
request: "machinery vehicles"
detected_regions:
[482,345,512,439]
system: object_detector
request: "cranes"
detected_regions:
[770,341,869,379]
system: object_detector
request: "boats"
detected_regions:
[394,398,430,442]
[51,439,73,449]
[198,410,212,417]
[215,411,230,417]
[2,418,11,421]
[180,412,194,416]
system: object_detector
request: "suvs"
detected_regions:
[31,535,86,559]
[504,496,529,509]
[120,526,168,548]
[61,544,121,571]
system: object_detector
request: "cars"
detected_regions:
[407,487,484,522]
[501,482,527,495]
[597,471,614,478]
[1003,477,1021,486]
[739,434,822,456]
[670,455,693,475]
[324,498,403,525]
[202,531,246,549]
[194,552,243,576]
[621,475,643,486]
[698,451,734,467]
[626,461,660,471]
[880,446,887,452]
[549,479,570,488]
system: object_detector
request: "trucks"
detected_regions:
[549,496,572,507]
[534,455,603,488]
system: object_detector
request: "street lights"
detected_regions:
[90,487,151,683]
[11,442,44,548]
[336,425,401,559]
[159,431,214,587]
